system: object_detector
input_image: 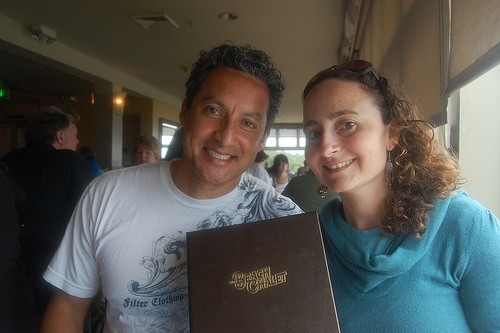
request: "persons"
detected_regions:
[0,106,184,333]
[281,169,343,215]
[40,44,305,333]
[301,60,500,333]
[245,148,309,195]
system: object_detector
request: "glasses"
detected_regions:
[303,60,380,98]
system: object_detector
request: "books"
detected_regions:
[186,211,342,333]
